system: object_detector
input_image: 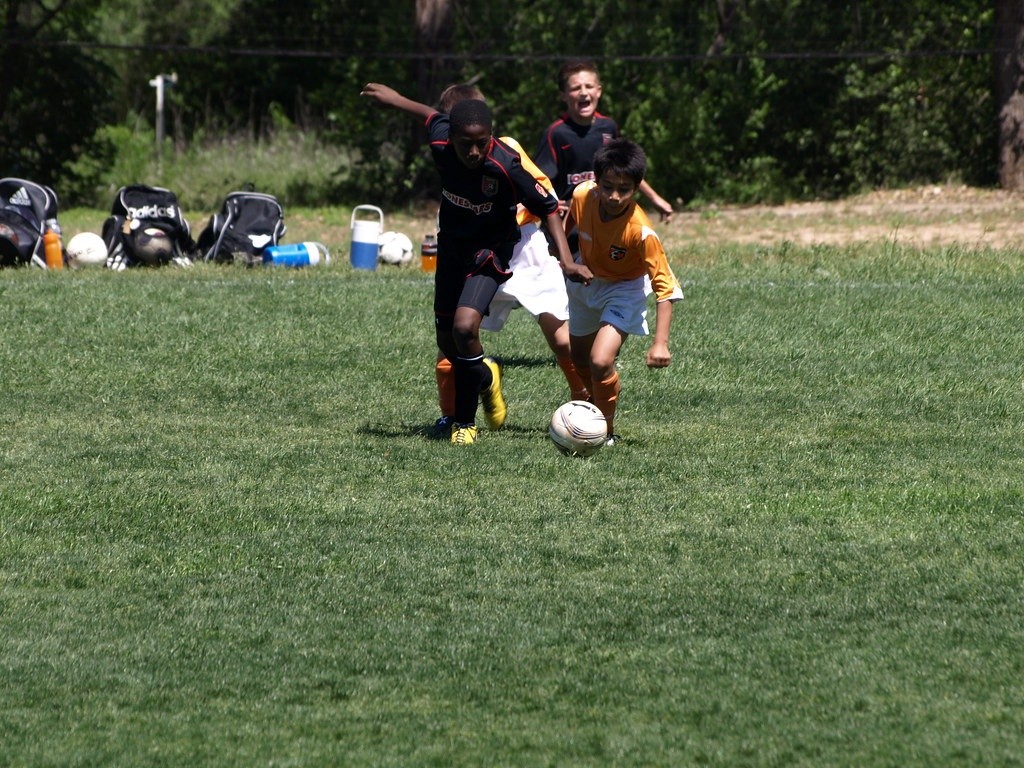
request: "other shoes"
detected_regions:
[436,414,453,430]
[603,434,620,447]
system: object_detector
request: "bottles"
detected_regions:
[46,218,63,250]
[421,234,437,274]
[44,230,63,272]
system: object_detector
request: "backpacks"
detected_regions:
[0,177,58,270]
[196,192,287,265]
[101,184,195,272]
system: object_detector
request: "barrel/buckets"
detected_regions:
[263,242,330,269]
[349,205,383,272]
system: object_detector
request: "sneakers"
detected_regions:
[450,422,478,445]
[478,357,507,432]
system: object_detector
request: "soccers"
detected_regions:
[133,229,172,264]
[377,231,414,268]
[66,233,109,271]
[550,401,607,458]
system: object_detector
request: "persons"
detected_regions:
[557,139,684,447]
[358,59,683,447]
